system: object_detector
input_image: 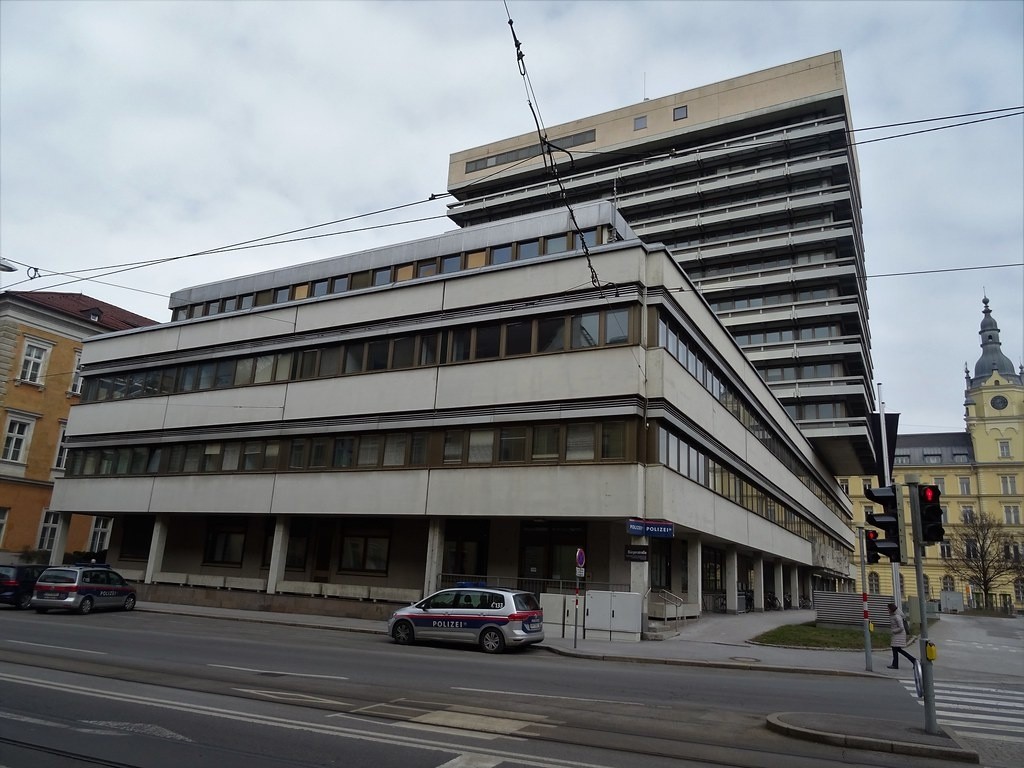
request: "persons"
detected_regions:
[888,603,916,669]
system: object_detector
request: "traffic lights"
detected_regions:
[864,529,880,563]
[918,484,945,542]
[864,484,907,565]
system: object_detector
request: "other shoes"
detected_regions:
[887,665,897,669]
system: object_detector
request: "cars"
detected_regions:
[386,587,544,652]
[32,564,138,613]
[0,563,53,611]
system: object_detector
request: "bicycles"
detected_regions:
[715,589,812,613]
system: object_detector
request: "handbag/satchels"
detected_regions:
[902,619,910,635]
